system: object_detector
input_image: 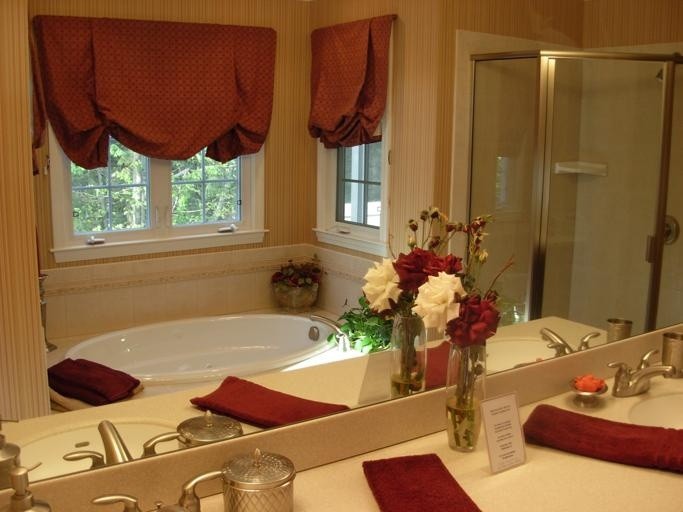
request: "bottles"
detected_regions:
[176,410,243,451]
[223,448,298,512]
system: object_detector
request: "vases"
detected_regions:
[272,282,319,311]
[391,315,428,398]
[444,340,486,451]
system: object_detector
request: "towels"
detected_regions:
[189,377,350,432]
[522,404,681,473]
[47,357,146,412]
[360,453,482,512]
[417,339,453,392]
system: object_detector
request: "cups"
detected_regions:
[606,317,633,346]
[661,333,683,379]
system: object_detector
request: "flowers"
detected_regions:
[411,217,520,449]
[329,207,464,390]
[272,252,323,290]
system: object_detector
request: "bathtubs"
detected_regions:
[66,314,343,384]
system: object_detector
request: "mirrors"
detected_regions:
[1,3,683,490]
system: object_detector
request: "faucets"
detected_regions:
[310,314,348,342]
[99,420,134,466]
[631,364,676,384]
[540,326,572,354]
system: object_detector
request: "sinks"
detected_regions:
[485,336,553,370]
[629,391,682,429]
[15,419,173,479]
